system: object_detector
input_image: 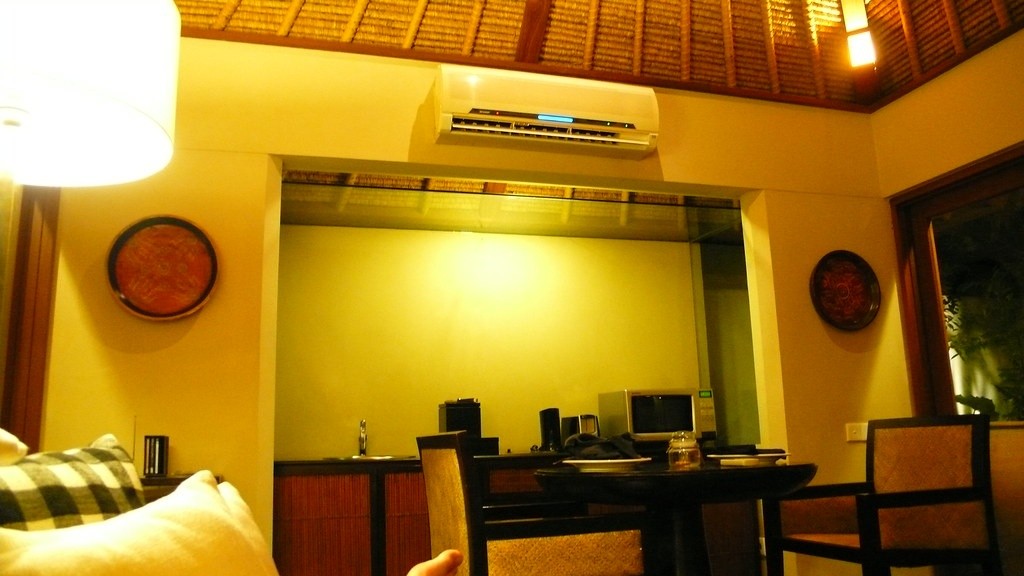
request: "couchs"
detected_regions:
[0,428,279,576]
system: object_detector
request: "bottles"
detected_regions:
[668,431,702,470]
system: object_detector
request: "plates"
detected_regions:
[562,457,651,471]
[706,453,791,464]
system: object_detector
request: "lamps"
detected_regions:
[0,1,181,187]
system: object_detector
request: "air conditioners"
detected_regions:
[434,63,660,160]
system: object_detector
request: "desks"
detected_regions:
[534,464,818,576]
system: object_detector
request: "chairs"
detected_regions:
[763,415,1003,576]
[416,430,661,576]
